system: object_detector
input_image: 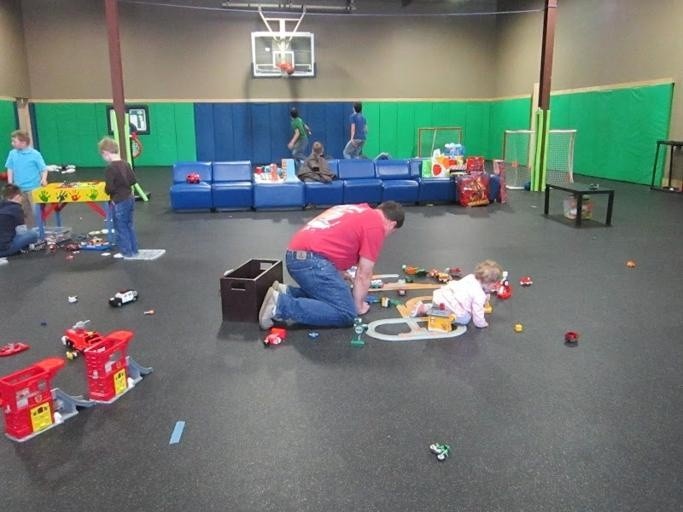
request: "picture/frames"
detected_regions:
[107,104,150,135]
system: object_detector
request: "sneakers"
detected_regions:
[272,280,287,321]
[259,286,281,330]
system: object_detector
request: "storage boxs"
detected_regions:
[220,259,282,322]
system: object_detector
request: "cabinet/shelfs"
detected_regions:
[650,141,683,192]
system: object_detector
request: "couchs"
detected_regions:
[339,160,383,204]
[212,161,252,213]
[299,160,343,206]
[412,161,455,205]
[170,162,211,211]
[375,160,419,204]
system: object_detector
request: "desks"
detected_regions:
[32,182,111,250]
[544,181,613,228]
[253,181,305,210]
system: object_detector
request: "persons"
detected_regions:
[5,130,48,226]
[312,141,325,157]
[258,200,406,331]
[287,107,310,160]
[409,259,504,329]
[0,184,46,257]
[97,137,138,259]
[343,101,367,160]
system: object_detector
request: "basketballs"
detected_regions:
[285,63,295,76]
